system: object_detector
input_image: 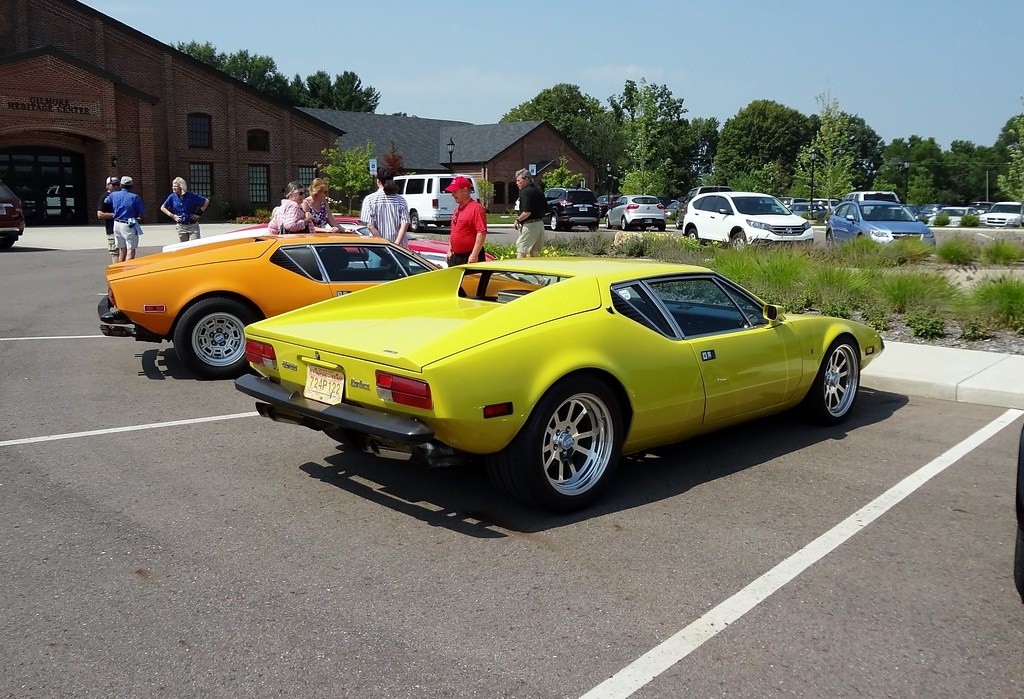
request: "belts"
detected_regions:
[179,221,194,225]
[526,219,542,223]
[115,220,128,224]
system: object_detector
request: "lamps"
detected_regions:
[111,156,118,167]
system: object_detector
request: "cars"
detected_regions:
[776,196,839,220]
[920,201,1024,229]
[843,191,900,202]
[604,194,666,231]
[46,184,75,223]
[0,180,25,249]
[597,195,620,218]
[682,192,815,252]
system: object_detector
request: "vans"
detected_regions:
[394,173,481,233]
[675,186,732,230]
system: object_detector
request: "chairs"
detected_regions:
[319,247,350,281]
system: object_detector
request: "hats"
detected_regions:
[374,167,394,179]
[120,176,134,185]
[106,176,120,185]
[445,176,475,194]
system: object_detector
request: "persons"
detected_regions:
[160,177,209,243]
[445,177,487,267]
[360,167,411,249]
[268,178,355,234]
[514,168,549,259]
[96,176,144,264]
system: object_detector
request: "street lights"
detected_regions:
[904,160,910,204]
[809,149,817,219]
[446,136,455,173]
[771,172,774,195]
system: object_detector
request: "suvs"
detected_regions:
[544,187,600,231]
[825,199,936,249]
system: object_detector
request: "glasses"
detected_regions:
[321,190,328,194]
[109,183,120,186]
[292,192,305,195]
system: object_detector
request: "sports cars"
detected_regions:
[234,256,886,514]
[160,227,450,270]
[226,218,496,262]
[98,232,543,382]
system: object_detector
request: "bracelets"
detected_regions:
[201,208,204,212]
[170,214,173,217]
[517,218,521,222]
